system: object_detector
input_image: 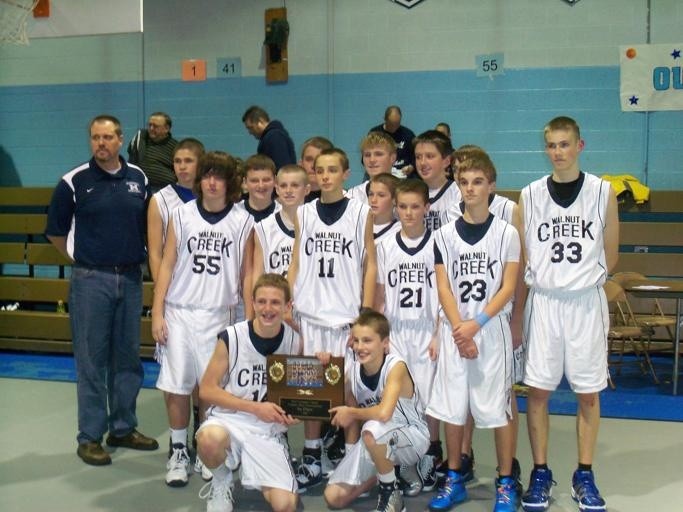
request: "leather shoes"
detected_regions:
[107,430,158,450]
[77,443,111,465]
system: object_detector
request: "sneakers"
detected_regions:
[166,443,190,487]
[494,458,520,512]
[373,484,404,512]
[207,469,234,511]
[522,468,553,510]
[195,454,213,480]
[399,448,473,510]
[570,468,606,511]
[294,427,344,493]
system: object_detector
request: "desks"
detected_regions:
[627,277,682,395]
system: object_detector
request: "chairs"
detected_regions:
[605,271,675,377]
[592,277,655,390]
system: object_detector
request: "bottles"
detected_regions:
[55,300,66,312]
